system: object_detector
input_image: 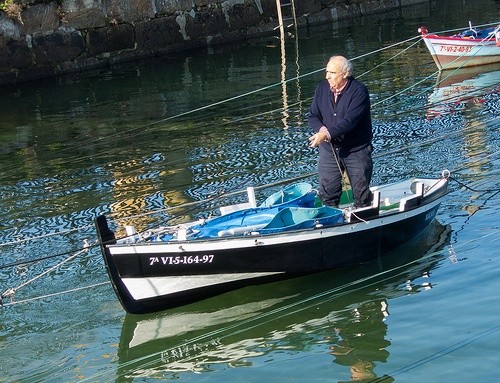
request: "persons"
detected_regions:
[308,55,374,212]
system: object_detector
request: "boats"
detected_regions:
[91,167,452,314]
[418,25,500,70]
